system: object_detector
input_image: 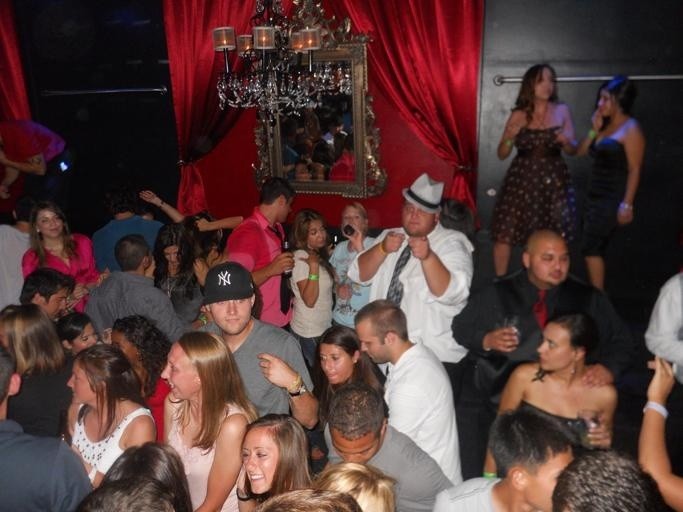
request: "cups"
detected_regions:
[576,410,604,451]
[501,313,525,340]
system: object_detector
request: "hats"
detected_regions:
[203,262,253,305]
[402,172,445,214]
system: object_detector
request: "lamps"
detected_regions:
[211,1,334,125]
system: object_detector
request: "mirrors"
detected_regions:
[248,1,389,202]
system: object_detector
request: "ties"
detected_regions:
[532,290,548,329]
[267,226,291,315]
[387,241,411,306]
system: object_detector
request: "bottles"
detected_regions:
[344,224,353,237]
[282,239,292,279]
